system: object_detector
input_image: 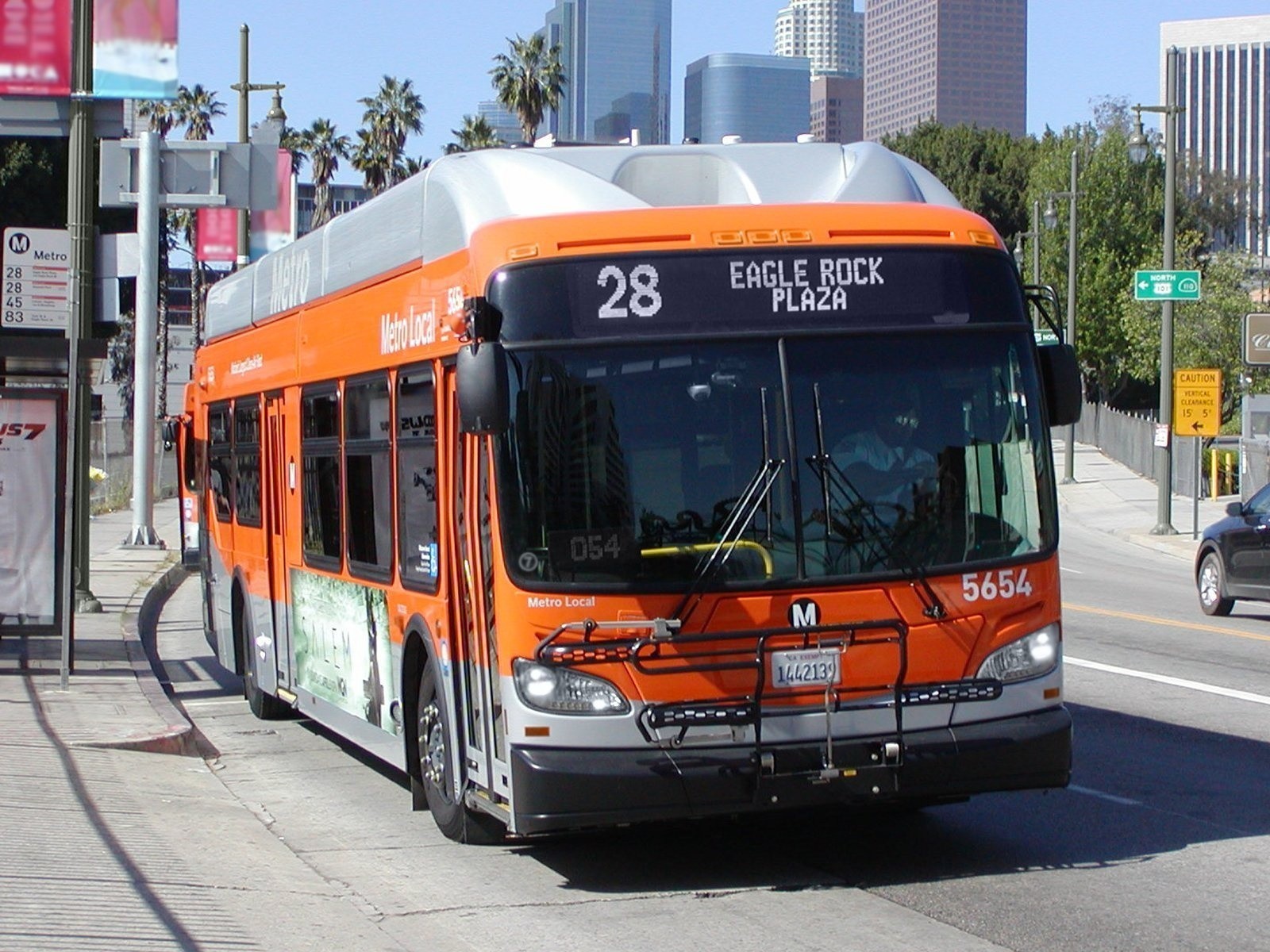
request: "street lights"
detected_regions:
[230,22,288,271]
[1013,200,1042,330]
[1042,149,1083,485]
[1125,44,1182,537]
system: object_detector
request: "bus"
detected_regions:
[192,129,1085,845]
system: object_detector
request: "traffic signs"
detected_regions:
[1135,270,1201,301]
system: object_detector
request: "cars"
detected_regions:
[1195,481,1270,616]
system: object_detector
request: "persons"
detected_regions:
[830,394,939,529]
